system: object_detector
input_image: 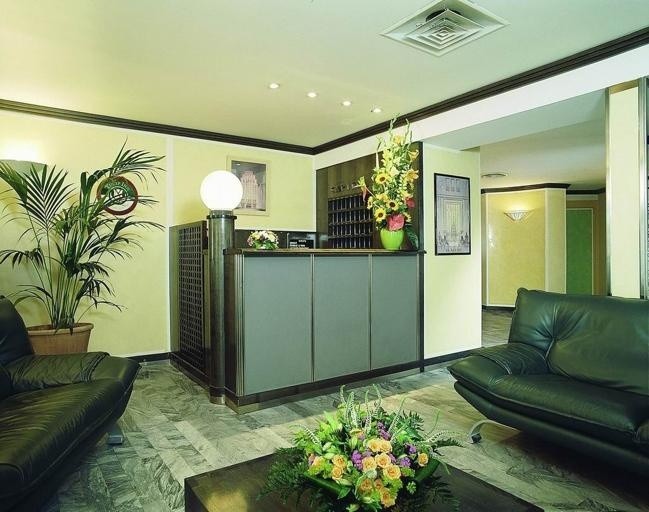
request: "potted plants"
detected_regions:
[1,133,165,356]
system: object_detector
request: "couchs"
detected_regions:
[0,296,141,512]
[451,286,648,483]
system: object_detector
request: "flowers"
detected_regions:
[249,230,279,250]
[253,383,468,512]
[353,113,420,251]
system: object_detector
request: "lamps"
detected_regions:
[503,209,532,223]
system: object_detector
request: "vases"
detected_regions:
[380,224,404,251]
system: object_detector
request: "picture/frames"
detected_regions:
[226,156,268,216]
[434,173,471,255]
